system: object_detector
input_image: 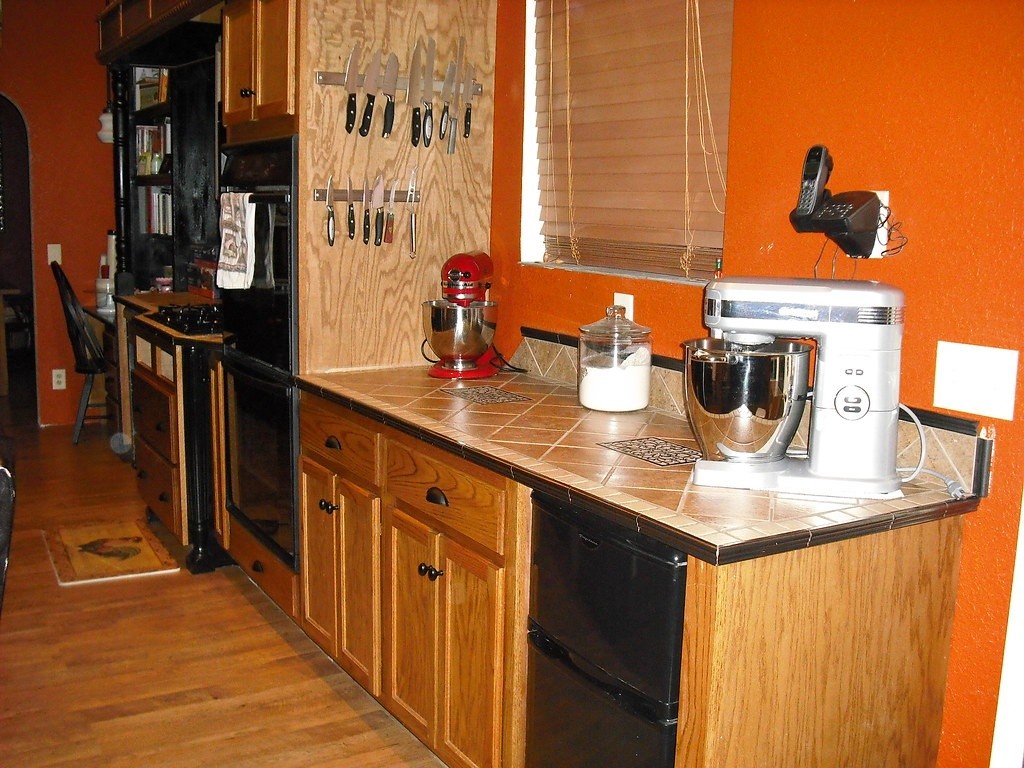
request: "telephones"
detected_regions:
[790,143,880,259]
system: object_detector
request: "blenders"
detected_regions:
[421,249,498,379]
[678,277,907,501]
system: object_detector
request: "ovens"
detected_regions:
[521,489,686,767]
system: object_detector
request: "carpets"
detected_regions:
[42,517,182,586]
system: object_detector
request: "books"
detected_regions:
[137,186,173,235]
[135,124,172,176]
[132,67,168,110]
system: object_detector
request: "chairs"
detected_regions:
[50,260,108,445]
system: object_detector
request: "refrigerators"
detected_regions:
[217,132,300,577]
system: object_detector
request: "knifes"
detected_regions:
[324,164,418,259]
[341,36,475,155]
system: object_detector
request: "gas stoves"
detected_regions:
[136,283,280,341]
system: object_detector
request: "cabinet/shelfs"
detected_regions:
[95,0,964,768]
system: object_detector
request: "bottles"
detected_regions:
[576,303,651,413]
